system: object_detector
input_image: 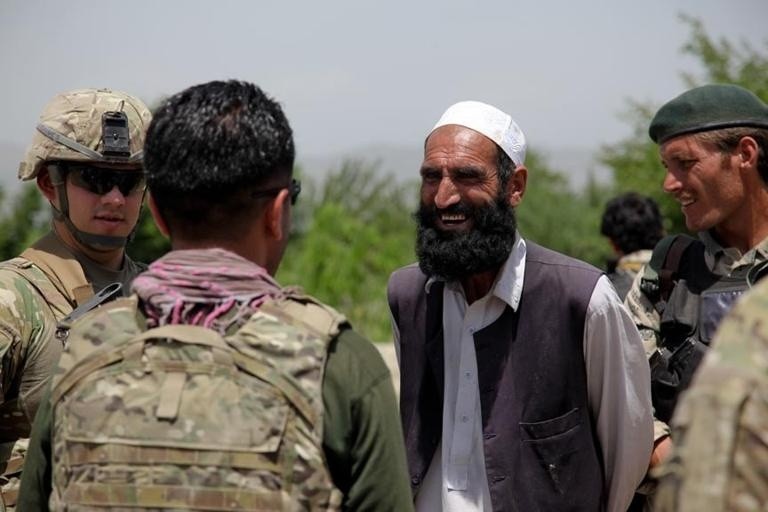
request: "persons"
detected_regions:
[386,100,654,512]
[16,78,417,512]
[650,275,768,512]
[600,191,664,303]
[623,83,768,512]
[0,90,154,512]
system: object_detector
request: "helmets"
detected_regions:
[18,88,153,181]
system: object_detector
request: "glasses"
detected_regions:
[70,164,148,195]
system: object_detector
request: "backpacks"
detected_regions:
[49,294,347,512]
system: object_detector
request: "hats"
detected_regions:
[650,84,768,144]
[425,100,526,167]
